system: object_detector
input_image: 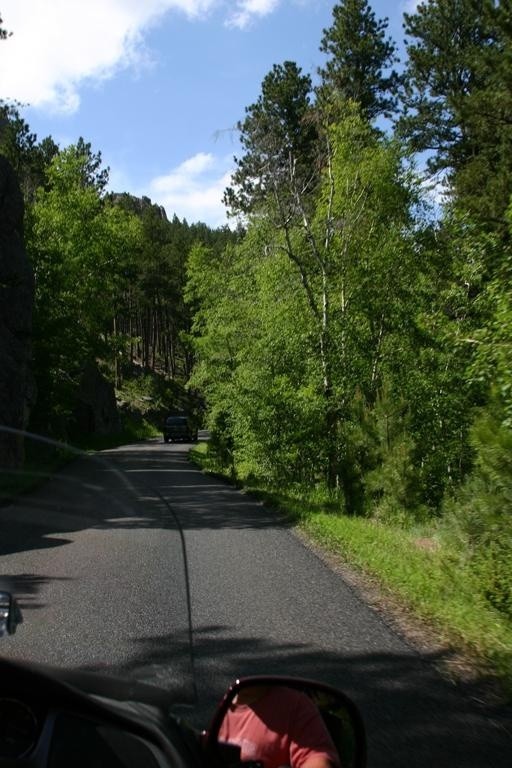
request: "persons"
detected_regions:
[217,686,341,768]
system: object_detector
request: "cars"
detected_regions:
[163,412,198,443]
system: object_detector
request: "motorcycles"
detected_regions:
[0,428,367,768]
